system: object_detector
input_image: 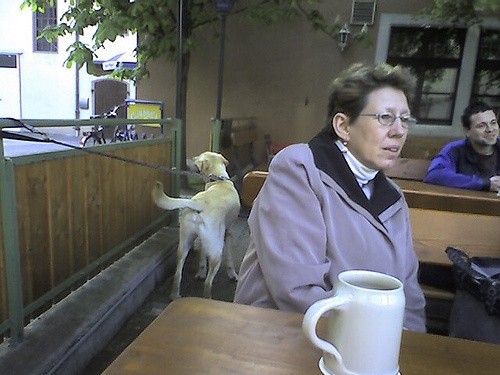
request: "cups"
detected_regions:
[301,270,406,375]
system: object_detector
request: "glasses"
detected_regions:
[345,112,417,129]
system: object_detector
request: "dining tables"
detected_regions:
[101,297,499,375]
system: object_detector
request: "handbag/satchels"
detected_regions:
[445,247,500,344]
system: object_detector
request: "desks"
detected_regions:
[407,207,500,289]
[387,178,500,216]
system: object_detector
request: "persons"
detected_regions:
[232,61,429,336]
[424,100,500,198]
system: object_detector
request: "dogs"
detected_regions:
[151,150,238,301]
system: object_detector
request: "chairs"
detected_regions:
[241,172,268,208]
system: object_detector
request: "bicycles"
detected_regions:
[80,104,126,147]
